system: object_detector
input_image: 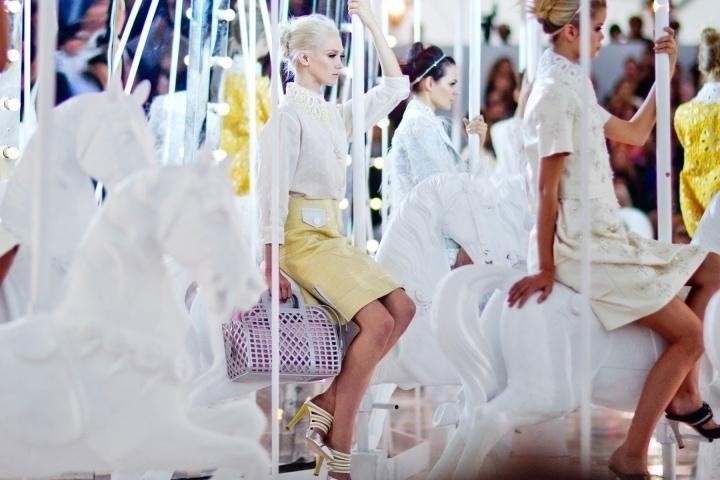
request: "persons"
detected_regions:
[23,0,191,106]
[390,41,488,269]
[590,16,679,234]
[508,0,720,480]
[478,22,520,139]
[258,0,417,480]
[673,26,720,239]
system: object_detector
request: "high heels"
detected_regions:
[286,396,335,464]
[314,449,351,480]
[666,402,720,449]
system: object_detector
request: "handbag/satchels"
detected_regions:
[221,285,340,384]
